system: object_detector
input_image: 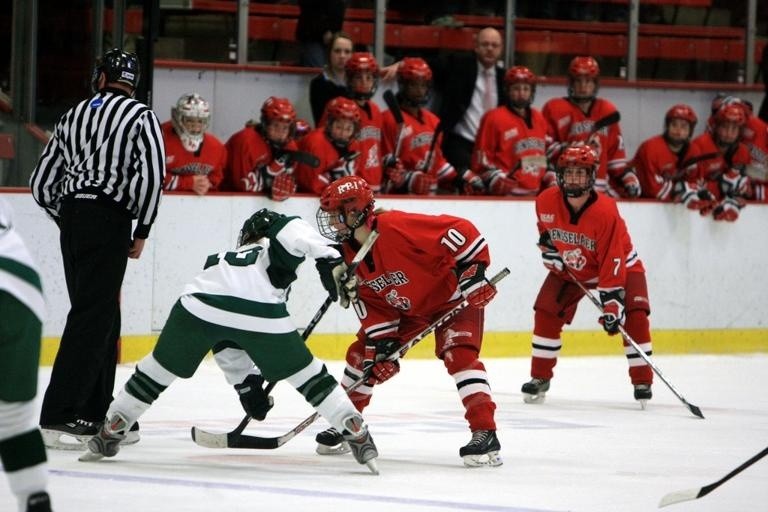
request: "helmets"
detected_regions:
[665,96,749,127]
[290,118,310,134]
[92,49,139,98]
[317,176,374,242]
[502,56,600,84]
[172,94,210,152]
[326,52,432,123]
[261,96,297,125]
[237,208,286,249]
[555,145,600,196]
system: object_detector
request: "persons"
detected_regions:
[0,209,54,512]
[316,175,502,458]
[521,144,653,399]
[161,2,768,221]
[87,207,378,464]
[29,47,167,436]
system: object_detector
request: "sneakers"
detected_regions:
[316,427,352,445]
[459,429,504,457]
[89,410,131,457]
[40,414,139,436]
[522,377,549,394]
[341,415,377,464]
[634,384,653,401]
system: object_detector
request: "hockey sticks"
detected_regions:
[586,110,619,145]
[423,113,456,173]
[674,151,720,182]
[191,228,381,449]
[265,147,320,193]
[564,265,704,418]
[383,89,405,194]
[658,445,768,506]
[218,269,511,449]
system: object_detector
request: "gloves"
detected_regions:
[314,244,358,308]
[540,247,566,272]
[363,337,400,388]
[405,161,556,196]
[598,290,627,335]
[621,169,642,198]
[262,141,300,201]
[328,149,360,180]
[456,260,496,309]
[674,170,767,221]
[382,154,406,187]
[235,373,273,421]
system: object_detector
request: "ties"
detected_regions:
[483,69,493,112]
